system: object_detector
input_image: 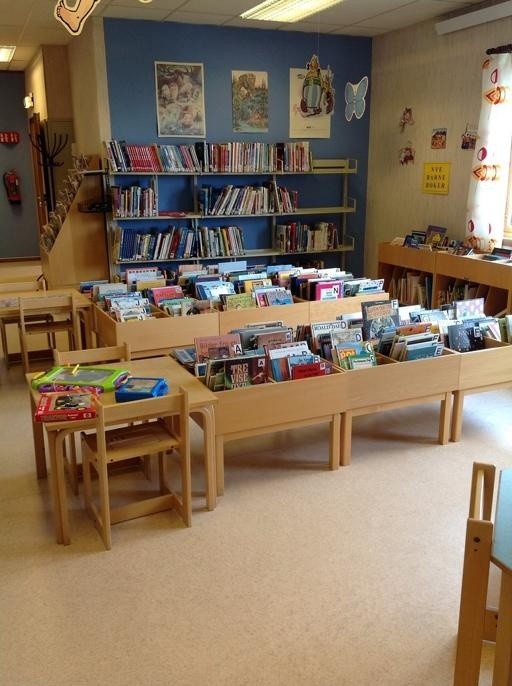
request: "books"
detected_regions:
[385,225,512,317]
[79,139,385,323]
[170,298,511,392]
[38,152,88,291]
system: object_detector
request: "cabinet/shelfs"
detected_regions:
[371,239,512,338]
[101,155,360,265]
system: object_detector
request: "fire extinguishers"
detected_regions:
[3,168,21,204]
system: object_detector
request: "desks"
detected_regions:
[22,356,219,546]
[0,288,93,370]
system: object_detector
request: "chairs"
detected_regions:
[75,383,190,551]
[19,298,82,373]
[51,339,140,495]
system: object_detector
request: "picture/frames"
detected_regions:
[153,58,207,140]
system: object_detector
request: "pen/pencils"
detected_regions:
[72,363,80,374]
